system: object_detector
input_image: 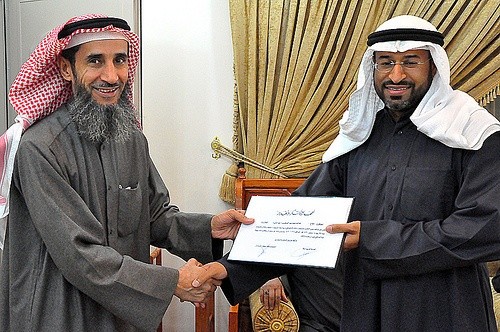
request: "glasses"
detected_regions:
[372,59,431,73]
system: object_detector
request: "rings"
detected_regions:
[264,291,269,295]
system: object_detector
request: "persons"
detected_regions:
[259,256,342,332]
[0,14,254,332]
[180,15,500,332]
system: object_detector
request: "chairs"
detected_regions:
[195,167,306,332]
